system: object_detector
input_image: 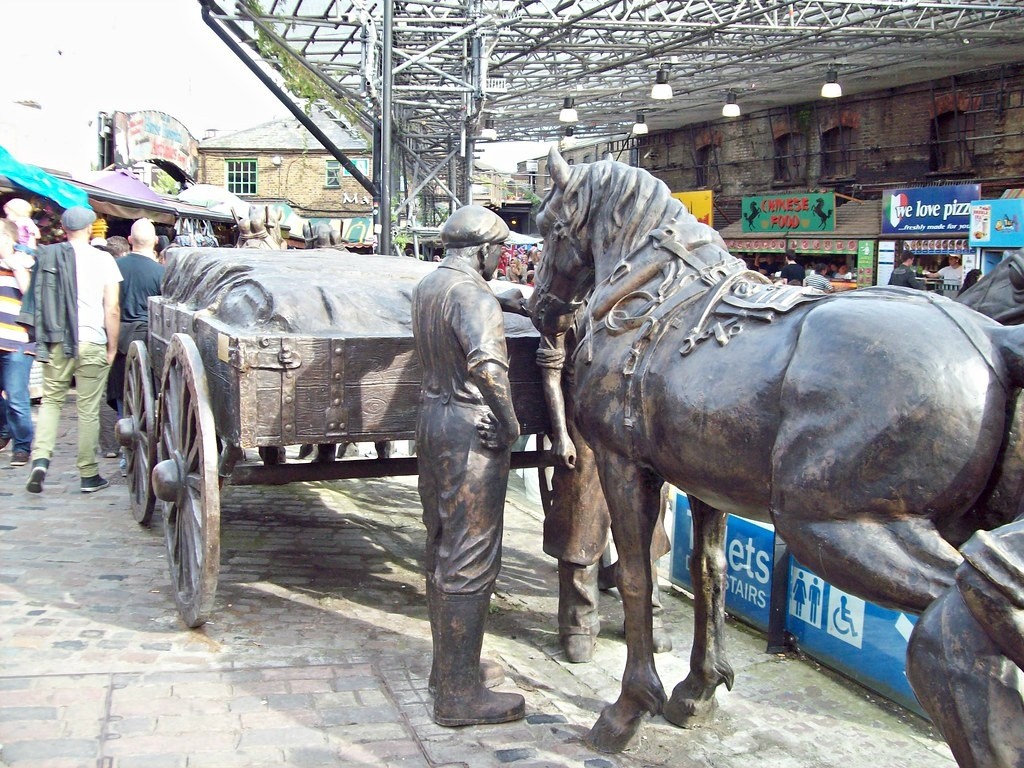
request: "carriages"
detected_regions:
[115,205,558,628]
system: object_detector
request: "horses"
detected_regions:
[232,205,350,253]
[526,147,1024,755]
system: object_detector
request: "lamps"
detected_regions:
[722,91,740,117]
[651,70,672,99]
[482,120,496,140]
[822,71,842,98]
[562,129,577,145]
[633,115,648,134]
[560,97,578,122]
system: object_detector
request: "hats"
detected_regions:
[61,207,96,231]
[3,198,33,218]
[518,248,525,254]
[156,235,169,251]
[949,254,962,260]
[91,237,108,250]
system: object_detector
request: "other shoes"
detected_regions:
[26,458,50,493]
[0,438,11,451]
[107,452,119,457]
[80,475,109,491]
[10,451,30,466]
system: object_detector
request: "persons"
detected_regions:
[23,206,124,494]
[494,245,543,288]
[738,250,984,299]
[536,282,672,662]
[411,204,526,727]
[91,219,191,476]
[0,198,41,465]
